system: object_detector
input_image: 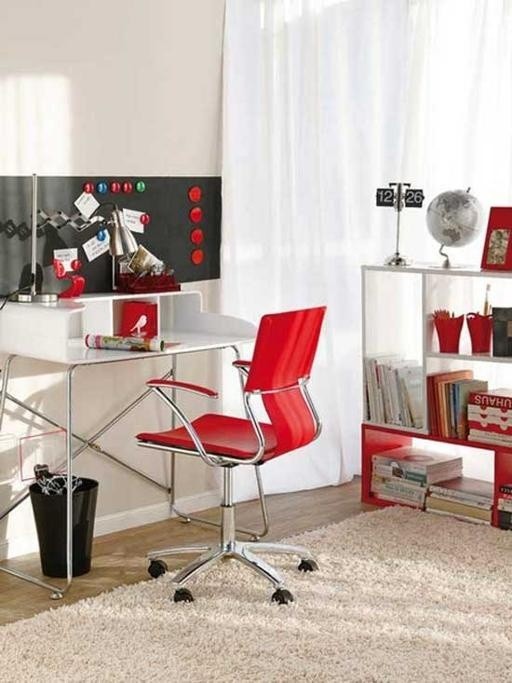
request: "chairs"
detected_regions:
[132,306,327,606]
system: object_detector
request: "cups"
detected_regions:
[433,311,466,353]
[467,312,495,353]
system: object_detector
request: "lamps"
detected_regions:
[31,200,140,257]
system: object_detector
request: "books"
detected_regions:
[367,356,511,530]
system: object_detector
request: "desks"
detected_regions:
[0,289,269,601]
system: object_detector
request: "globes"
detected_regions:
[425,187,485,268]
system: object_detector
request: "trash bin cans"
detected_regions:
[28,476,99,578]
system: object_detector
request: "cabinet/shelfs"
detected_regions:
[358,264,512,532]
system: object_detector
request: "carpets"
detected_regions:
[0,504,512,683]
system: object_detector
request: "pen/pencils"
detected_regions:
[432,308,455,319]
